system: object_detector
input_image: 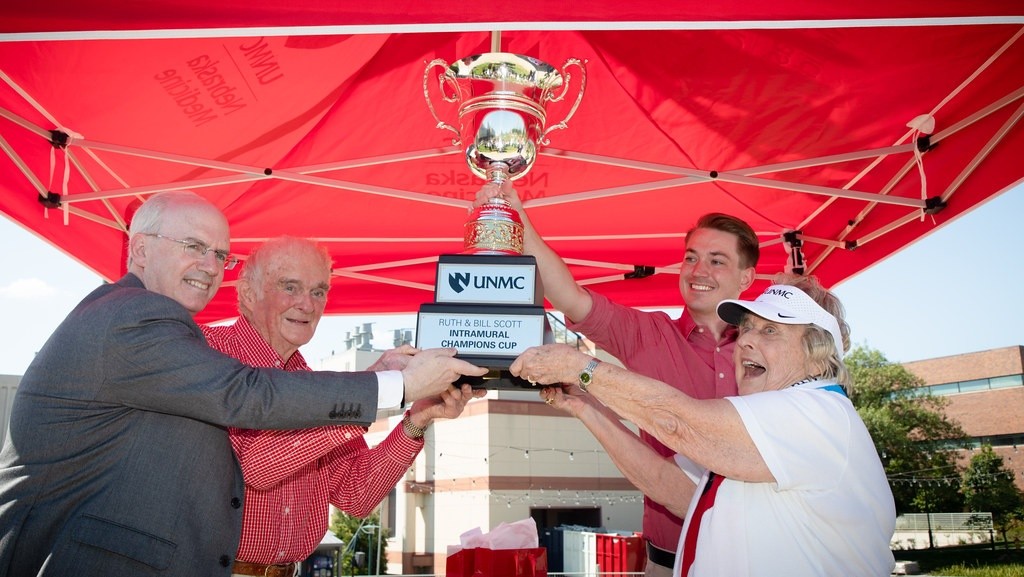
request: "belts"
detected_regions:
[645,545,674,568]
[235,561,298,577]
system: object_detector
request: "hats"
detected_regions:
[719,284,846,362]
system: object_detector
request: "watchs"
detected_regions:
[579,358,601,392]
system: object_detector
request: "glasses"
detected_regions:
[143,233,240,270]
[256,280,328,303]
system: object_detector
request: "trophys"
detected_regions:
[414,52,586,391]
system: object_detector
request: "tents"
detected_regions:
[1,0,1024,325]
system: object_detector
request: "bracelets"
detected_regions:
[404,410,428,437]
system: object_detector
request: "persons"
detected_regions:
[509,274,897,576]
[1,190,489,577]
[473,176,760,576]
[198,238,488,577]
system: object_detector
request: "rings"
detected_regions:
[546,399,553,405]
[528,376,536,386]
